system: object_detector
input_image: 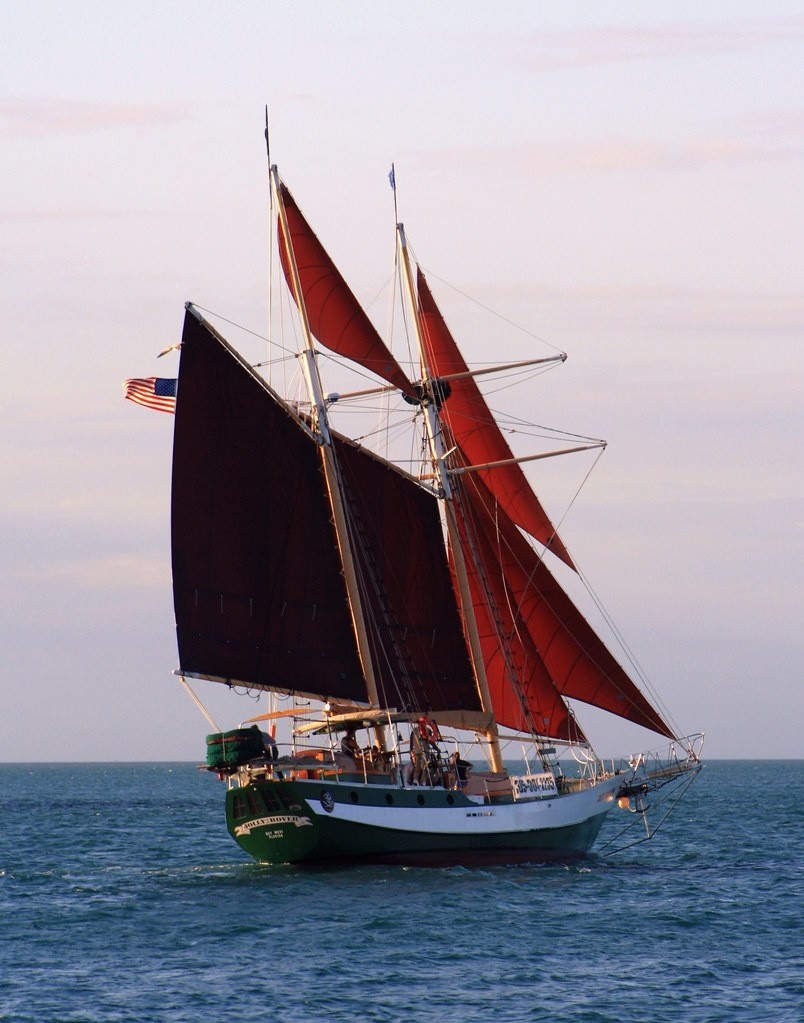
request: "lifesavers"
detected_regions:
[418,715,438,743]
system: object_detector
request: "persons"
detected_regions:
[340,729,363,760]
[251,724,285,781]
[411,718,473,790]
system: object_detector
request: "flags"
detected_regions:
[123,377,179,414]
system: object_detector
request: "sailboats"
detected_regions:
[123,105,706,873]
